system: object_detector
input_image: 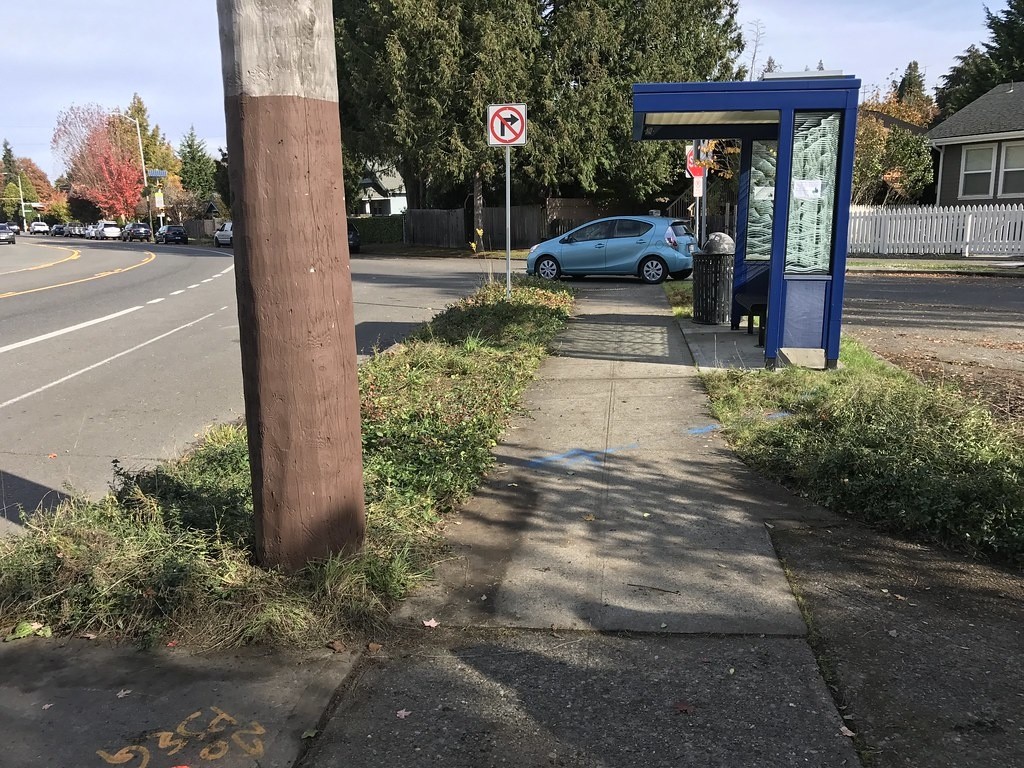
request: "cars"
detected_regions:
[50,225,65,236]
[85,224,99,239]
[6,222,20,235]
[346,218,360,253]
[526,216,699,285]
[94,223,121,240]
[154,225,188,245]
[213,221,233,246]
[0,223,15,244]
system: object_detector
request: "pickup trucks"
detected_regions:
[63,222,86,237]
[30,222,49,235]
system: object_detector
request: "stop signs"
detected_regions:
[687,147,707,178]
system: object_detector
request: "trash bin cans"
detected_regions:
[692,232,736,325]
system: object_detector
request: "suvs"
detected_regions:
[120,222,152,242]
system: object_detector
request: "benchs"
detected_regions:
[735,264,772,346]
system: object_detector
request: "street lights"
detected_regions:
[110,113,154,239]
[2,172,27,232]
